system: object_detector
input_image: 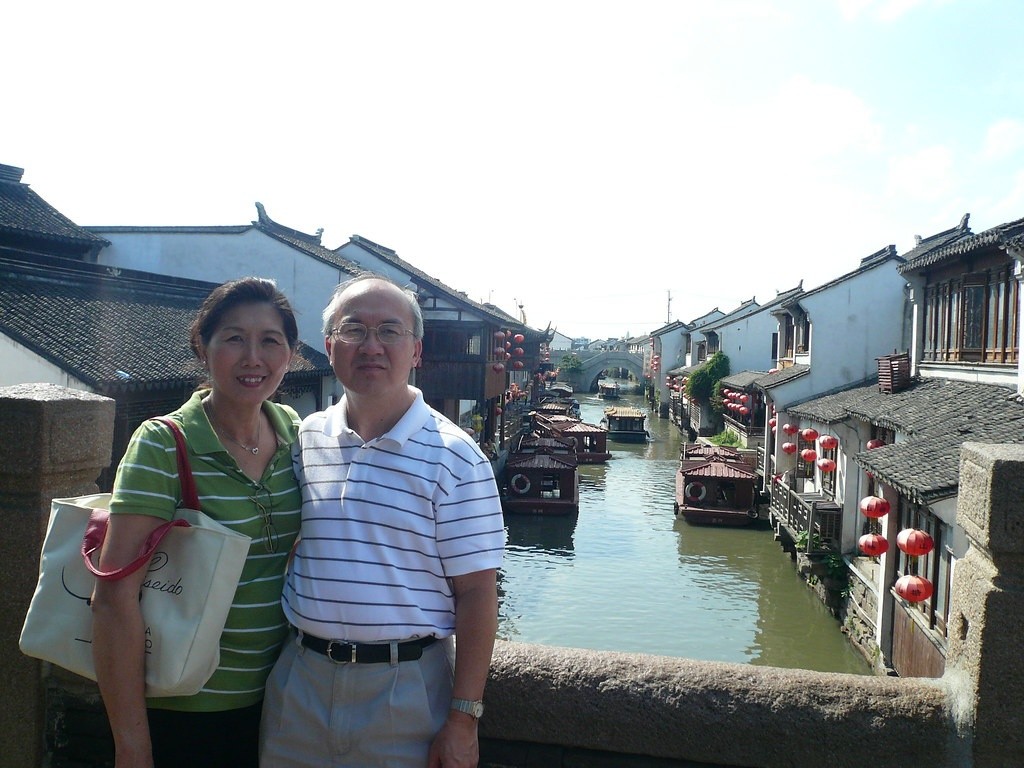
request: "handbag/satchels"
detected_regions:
[18,416,252,698]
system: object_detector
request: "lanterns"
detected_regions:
[463,428,474,437]
[506,330,512,360]
[513,334,524,369]
[471,414,483,432]
[769,368,779,375]
[649,338,653,347]
[894,528,934,601]
[867,439,886,450]
[768,403,776,434]
[666,376,698,405]
[781,424,797,455]
[493,329,505,374]
[545,352,550,361]
[496,406,502,416]
[505,366,561,407]
[800,428,818,462]
[857,495,889,557]
[723,389,749,415]
[650,355,659,372]
[817,435,837,472]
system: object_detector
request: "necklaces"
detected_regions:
[206,397,260,454]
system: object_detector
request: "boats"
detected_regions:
[674,441,759,526]
[601,406,646,443]
[519,383,613,464]
[502,451,579,515]
[597,384,620,400]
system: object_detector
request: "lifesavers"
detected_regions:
[567,436,578,450]
[511,474,530,494]
[747,509,759,519]
[685,481,707,502]
[674,502,678,515]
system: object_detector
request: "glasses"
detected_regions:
[329,323,417,345]
[252,488,280,555]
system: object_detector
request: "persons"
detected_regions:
[256,274,506,768]
[63,277,304,767]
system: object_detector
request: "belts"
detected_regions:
[293,625,438,664]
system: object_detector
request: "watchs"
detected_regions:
[449,697,484,722]
